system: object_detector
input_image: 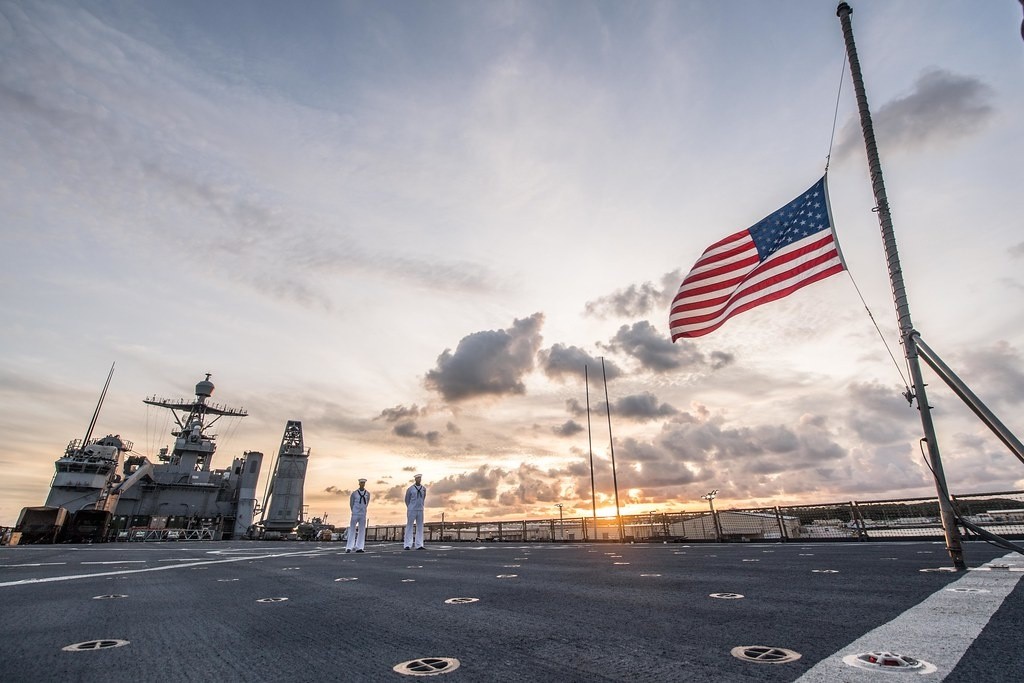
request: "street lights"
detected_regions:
[555,503,564,537]
[701,490,720,539]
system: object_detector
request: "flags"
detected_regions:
[669,172,848,343]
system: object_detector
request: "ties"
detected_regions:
[358,490,366,505]
[414,485,422,498]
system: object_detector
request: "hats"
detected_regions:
[358,479,367,483]
[415,474,422,479]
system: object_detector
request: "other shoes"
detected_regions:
[356,549,365,552]
[404,546,410,550]
[416,547,426,550]
[346,549,352,554]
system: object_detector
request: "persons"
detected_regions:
[345,478,370,553]
[404,474,426,550]
